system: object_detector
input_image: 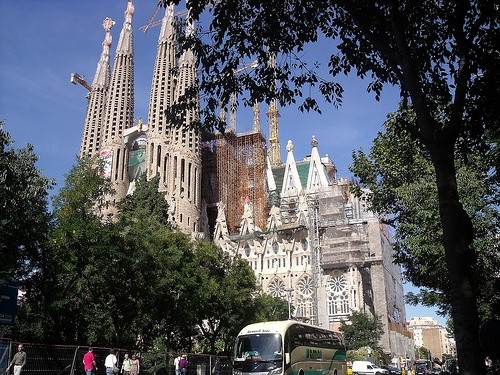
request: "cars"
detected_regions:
[383,365,402,375]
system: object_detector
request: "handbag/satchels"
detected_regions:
[181,368,186,373]
[113,365,120,373]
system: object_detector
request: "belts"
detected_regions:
[15,364,22,366]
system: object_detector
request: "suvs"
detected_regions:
[416,365,427,375]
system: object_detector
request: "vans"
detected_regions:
[351,359,388,375]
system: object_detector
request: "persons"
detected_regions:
[178,355,187,375]
[120,353,132,375]
[397,355,494,375]
[104,349,117,375]
[83,346,99,375]
[6,343,28,375]
[173,354,184,375]
[130,354,140,375]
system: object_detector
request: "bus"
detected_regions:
[233,320,347,375]
